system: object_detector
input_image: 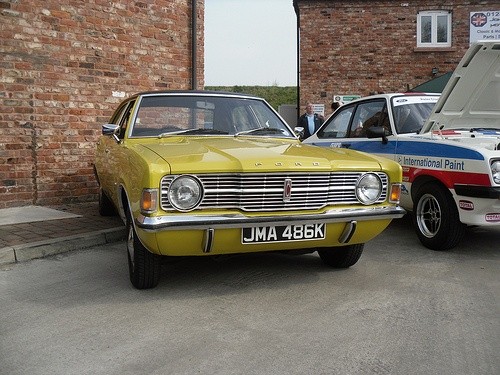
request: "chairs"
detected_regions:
[359,110,397,137]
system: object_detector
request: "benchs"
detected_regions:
[118,127,184,138]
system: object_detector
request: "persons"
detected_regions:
[331,105,364,138]
[296,101,325,143]
[323,102,340,123]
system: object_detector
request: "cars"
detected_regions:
[302,40,500,252]
[92,90,406,291]
